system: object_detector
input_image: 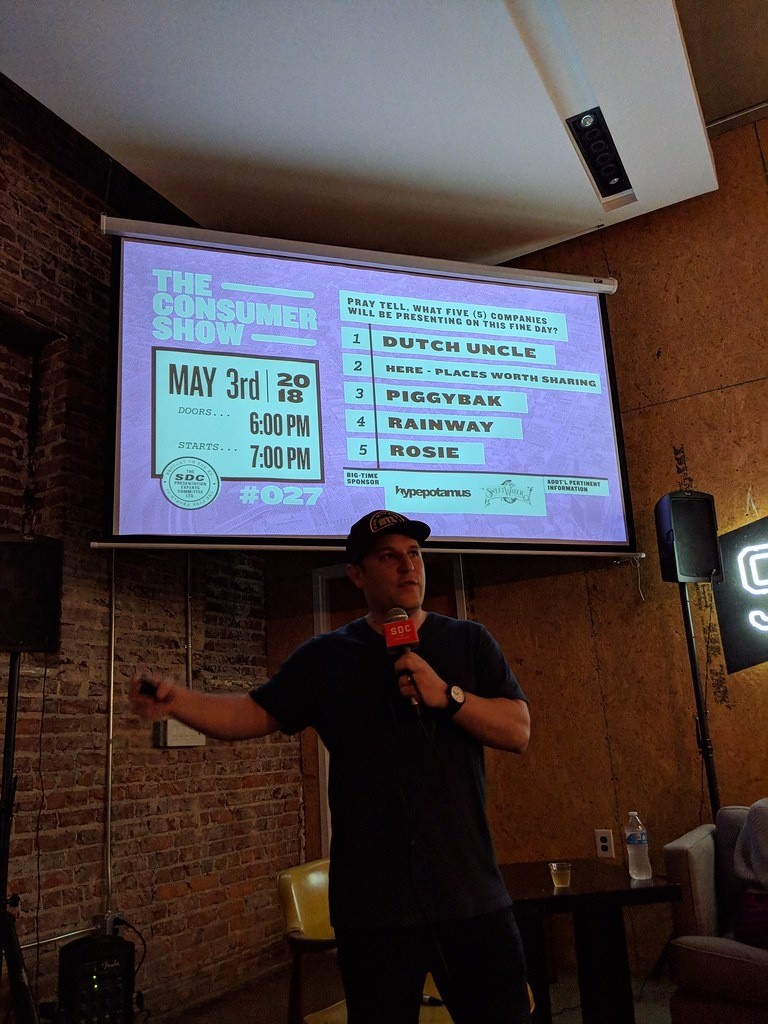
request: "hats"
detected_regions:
[347,510,432,566]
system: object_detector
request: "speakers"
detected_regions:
[58,933,136,1024]
[655,489,724,584]
[0,533,65,654]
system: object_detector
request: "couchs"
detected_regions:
[658,806,768,1024]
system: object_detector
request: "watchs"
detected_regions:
[438,680,467,719]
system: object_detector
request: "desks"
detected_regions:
[495,857,683,1024]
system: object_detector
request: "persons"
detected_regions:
[127,509,532,1024]
[732,795,768,950]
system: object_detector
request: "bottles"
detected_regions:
[625,811,652,880]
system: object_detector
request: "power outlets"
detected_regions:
[594,829,615,858]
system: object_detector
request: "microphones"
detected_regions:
[382,608,421,718]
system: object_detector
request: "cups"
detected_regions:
[548,863,572,888]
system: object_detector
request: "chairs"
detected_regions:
[276,857,336,1024]
